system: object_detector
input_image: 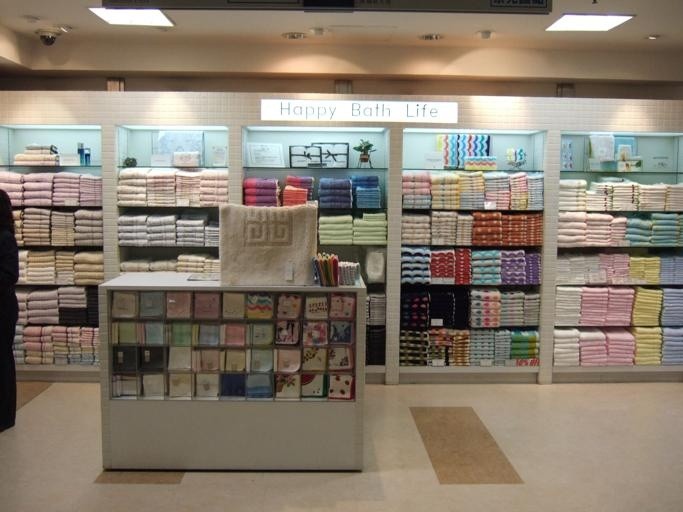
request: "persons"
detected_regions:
[0,188,18,435]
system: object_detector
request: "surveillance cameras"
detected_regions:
[35,27,59,46]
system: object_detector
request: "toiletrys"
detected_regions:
[84,148,90,165]
[77,142,84,165]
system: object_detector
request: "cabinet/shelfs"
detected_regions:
[97,271,364,470]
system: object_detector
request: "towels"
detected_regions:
[402,134,540,370]
[242,140,383,365]
[118,150,228,279]
[110,289,357,399]
[556,133,682,370]
[1,141,102,369]
[219,201,317,290]
